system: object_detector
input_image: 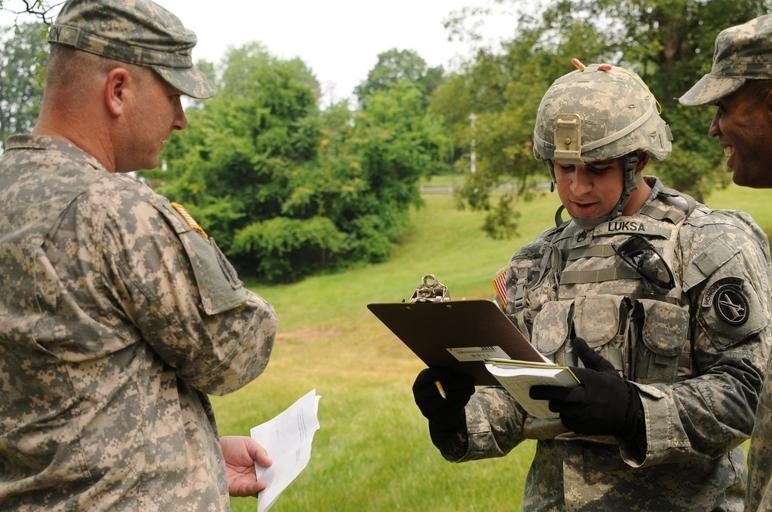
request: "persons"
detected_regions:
[0,0,279,512]
[679,14,772,512]
[411,59,772,512]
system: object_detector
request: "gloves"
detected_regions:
[528,336,640,442]
[412,362,477,430]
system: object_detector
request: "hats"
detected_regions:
[677,12,771,107]
[45,0,218,100]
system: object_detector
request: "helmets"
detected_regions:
[531,62,676,166]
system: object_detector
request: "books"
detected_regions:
[483,357,582,420]
